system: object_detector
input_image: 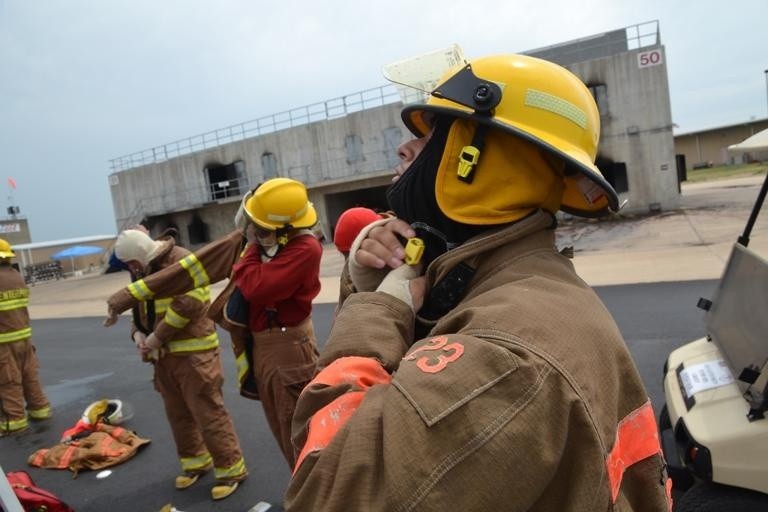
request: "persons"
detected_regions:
[284,51,673,512]
[104,175,324,475]
[0,239,52,436]
[115,227,248,501]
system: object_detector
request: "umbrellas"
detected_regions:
[51,246,103,272]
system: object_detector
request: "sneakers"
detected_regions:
[211,480,238,500]
[175,470,199,489]
[0,426,27,437]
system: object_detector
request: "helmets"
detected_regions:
[82,398,134,426]
[0,239,16,259]
[400,43,621,219]
[234,177,318,248]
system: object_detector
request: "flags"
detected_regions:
[8,176,17,188]
